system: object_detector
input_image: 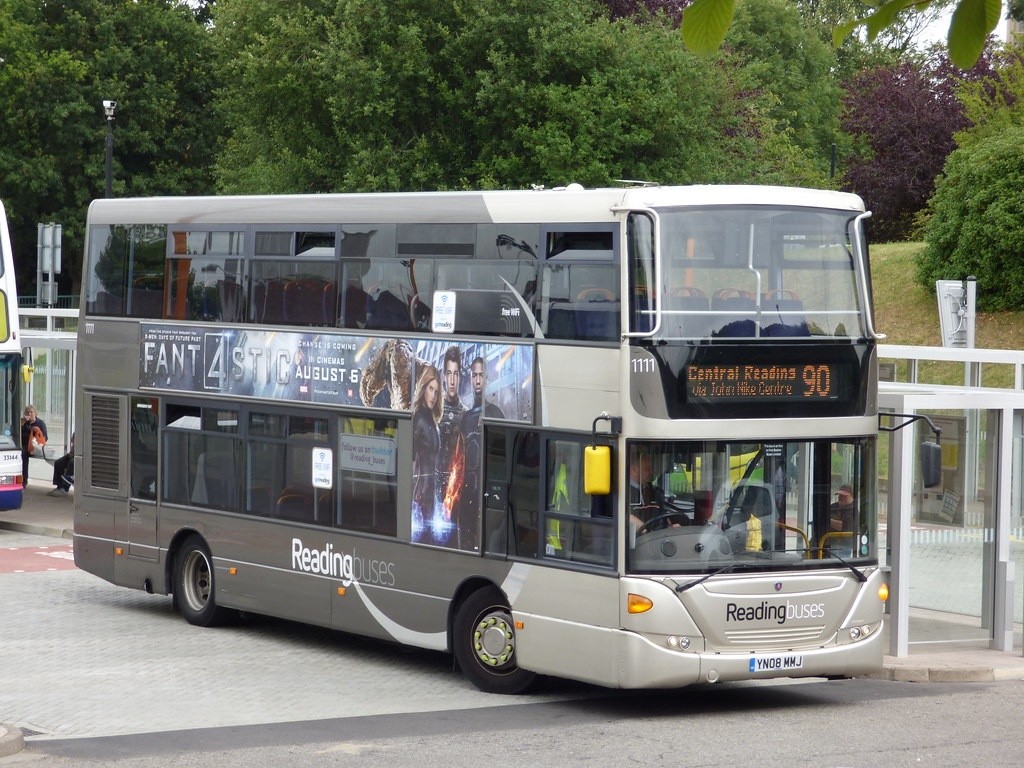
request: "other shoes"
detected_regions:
[53,489,70,497]
[46,487,59,495]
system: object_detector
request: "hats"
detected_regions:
[834,484,854,496]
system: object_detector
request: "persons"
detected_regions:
[10,404,48,489]
[46,432,75,498]
[412,346,504,554]
[829,484,858,558]
[618,452,680,533]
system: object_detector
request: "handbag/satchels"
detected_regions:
[28,426,47,453]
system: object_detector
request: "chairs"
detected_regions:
[129,267,810,342]
[132,462,396,540]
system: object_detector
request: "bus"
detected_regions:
[0,199,35,512]
[70,186,942,694]
